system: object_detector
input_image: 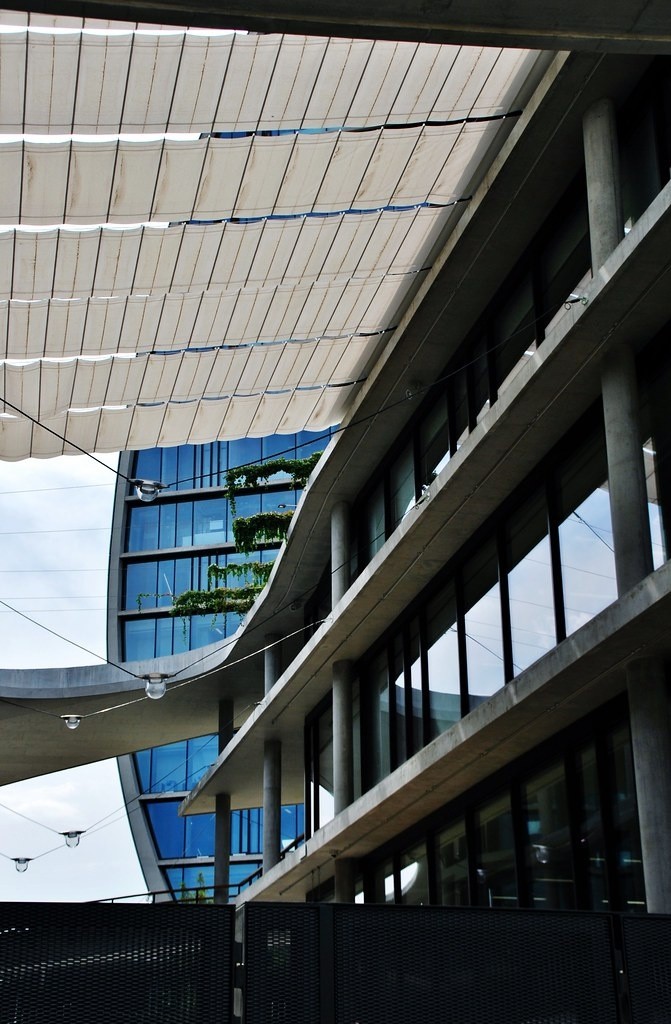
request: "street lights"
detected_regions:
[477,869,492,908]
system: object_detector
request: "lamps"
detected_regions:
[401,382,423,402]
[278,504,297,508]
